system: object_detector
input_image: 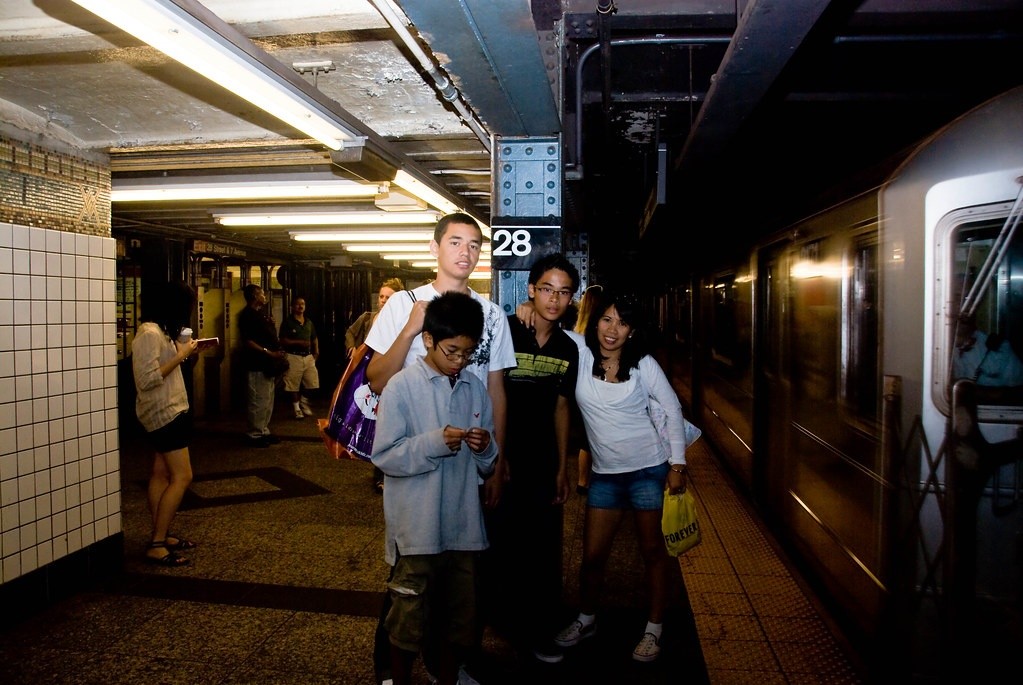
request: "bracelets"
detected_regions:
[314,352,319,355]
[671,466,687,475]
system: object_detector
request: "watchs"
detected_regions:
[264,348,268,353]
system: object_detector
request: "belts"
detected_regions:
[285,350,311,357]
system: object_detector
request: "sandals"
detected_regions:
[139,540,191,566]
[164,530,196,550]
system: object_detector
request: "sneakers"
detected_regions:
[633,632,661,661]
[554,620,596,647]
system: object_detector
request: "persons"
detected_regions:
[346,213,686,685]
[239,284,287,447]
[279,296,319,419]
[951,274,1019,393]
[132,280,216,565]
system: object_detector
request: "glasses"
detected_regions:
[533,287,572,296]
[433,338,473,362]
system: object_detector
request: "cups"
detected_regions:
[177,333,192,344]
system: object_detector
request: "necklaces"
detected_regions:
[603,355,618,382]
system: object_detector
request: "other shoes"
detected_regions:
[300,401,312,415]
[534,639,563,663]
[576,483,589,496]
[428,662,480,685]
[242,432,281,448]
[294,409,304,419]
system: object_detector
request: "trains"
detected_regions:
[636,84,1022,685]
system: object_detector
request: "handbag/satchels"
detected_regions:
[662,483,701,555]
[316,290,416,462]
[262,345,290,379]
